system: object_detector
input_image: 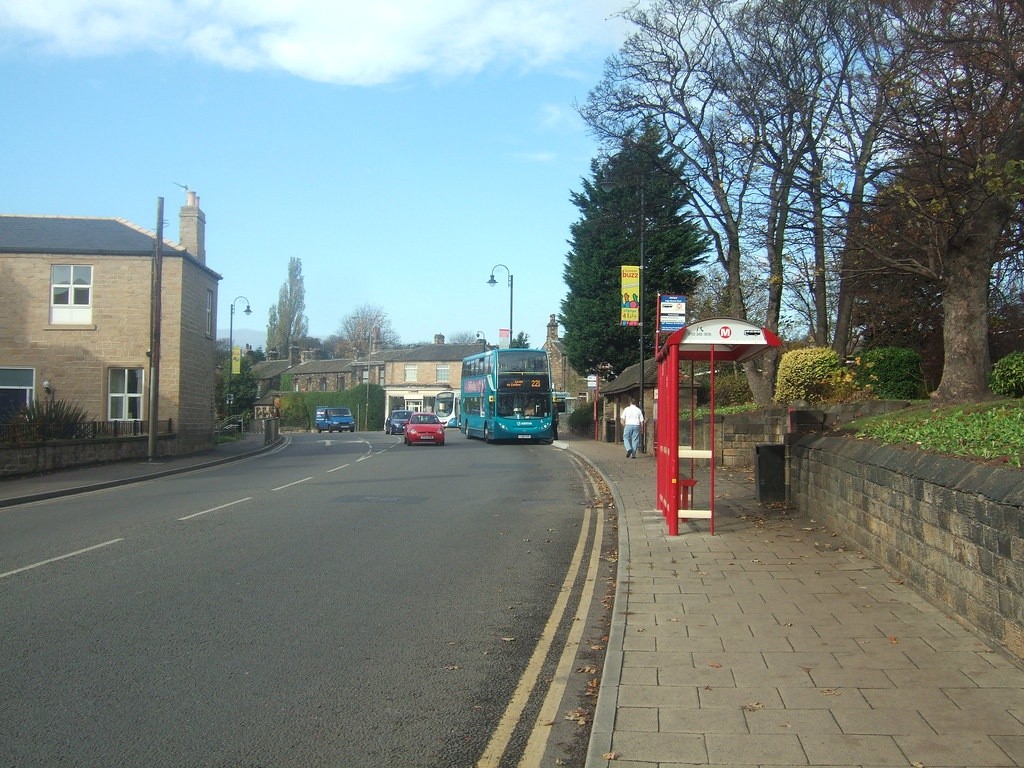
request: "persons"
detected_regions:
[552,402,559,440]
[525,401,534,416]
[621,399,644,458]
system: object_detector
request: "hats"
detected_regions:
[552,403,558,408]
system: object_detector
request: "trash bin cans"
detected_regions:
[607,420,615,442]
[755,444,786,503]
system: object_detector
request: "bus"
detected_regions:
[461,348,557,444]
[434,392,573,428]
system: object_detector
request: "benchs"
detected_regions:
[678,473,699,523]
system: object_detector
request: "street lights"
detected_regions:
[352,316,372,429]
[476,331,485,352]
[228,296,253,421]
[486,264,513,348]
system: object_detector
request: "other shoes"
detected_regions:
[626,450,632,458]
[632,456,635,458]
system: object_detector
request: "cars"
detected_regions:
[401,412,445,447]
[385,410,416,435]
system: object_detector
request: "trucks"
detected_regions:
[315,408,356,434]
